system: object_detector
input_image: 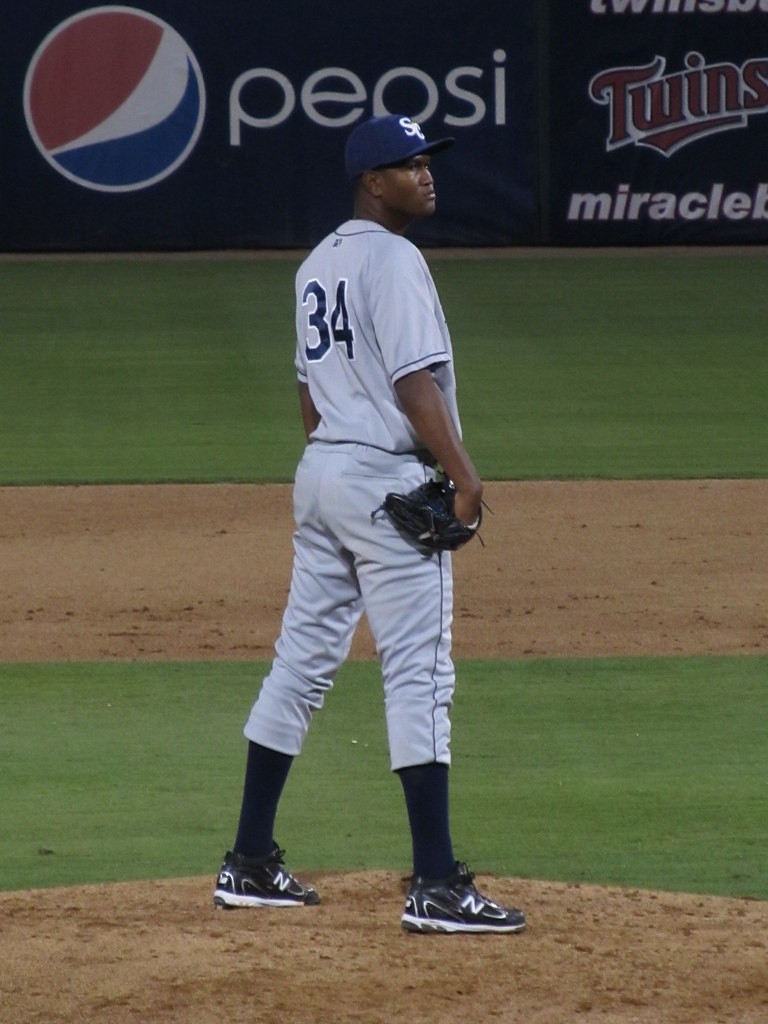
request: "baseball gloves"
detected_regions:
[371,470,493,559]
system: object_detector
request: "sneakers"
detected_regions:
[400,859,528,934]
[214,838,318,906]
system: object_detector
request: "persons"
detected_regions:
[213,115,526,934]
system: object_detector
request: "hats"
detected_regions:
[341,111,459,180]
